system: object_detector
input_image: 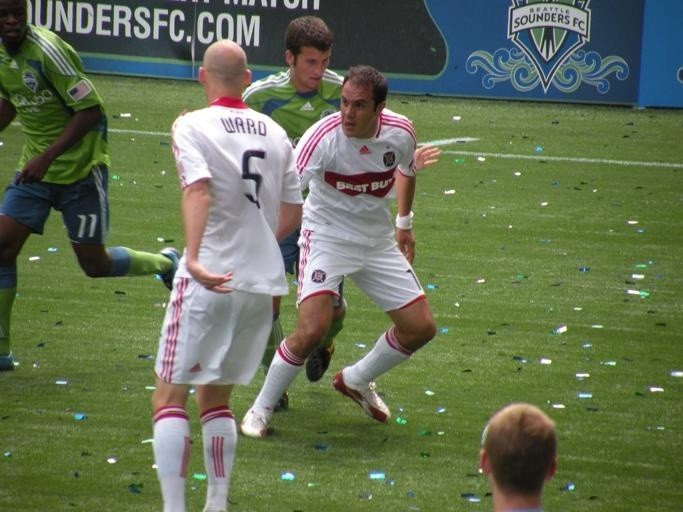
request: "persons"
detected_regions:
[0,1,183,372]
[240,16,442,410]
[239,65,442,439]
[151,39,303,512]
[478,402,557,512]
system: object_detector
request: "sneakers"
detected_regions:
[306,338,334,382]
[159,247,181,290]
[241,407,273,438]
[0,349,16,371]
[332,367,391,422]
[274,393,289,412]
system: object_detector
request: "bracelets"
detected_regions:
[395,211,415,231]
